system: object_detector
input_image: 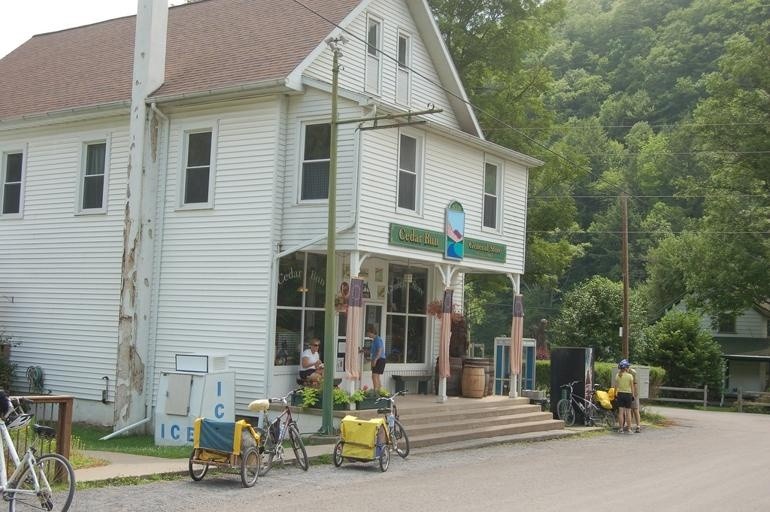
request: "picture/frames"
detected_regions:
[444,208,465,261]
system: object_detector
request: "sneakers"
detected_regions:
[618,425,641,434]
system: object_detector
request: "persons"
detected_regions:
[298,338,325,380]
[624,368,642,433]
[360,326,387,390]
[613,360,636,435]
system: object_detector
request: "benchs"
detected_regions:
[391,375,432,395]
[342,417,387,460]
[193,418,257,463]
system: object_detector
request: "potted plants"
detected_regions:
[291,386,392,411]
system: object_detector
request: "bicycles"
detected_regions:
[0,387,76,512]
[557,376,626,431]
[347,389,410,463]
[239,387,309,477]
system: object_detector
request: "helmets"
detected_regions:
[618,359,631,368]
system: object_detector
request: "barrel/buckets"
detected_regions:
[459,358,494,398]
